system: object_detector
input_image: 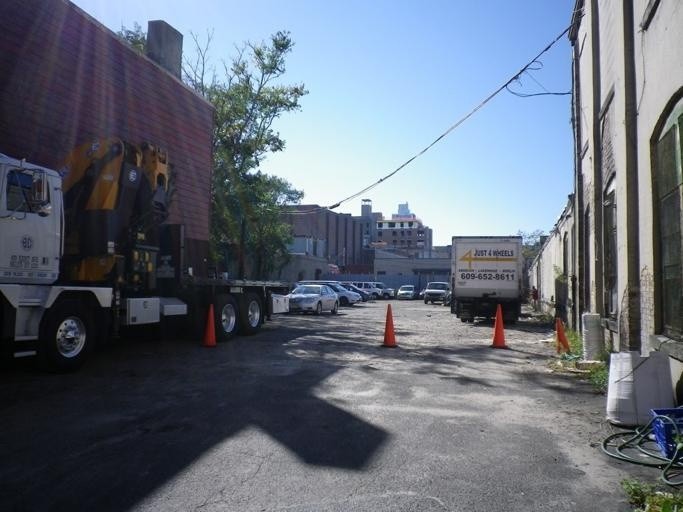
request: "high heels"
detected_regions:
[378,302,400,349]
[485,302,512,352]
[550,318,580,359]
[201,303,218,347]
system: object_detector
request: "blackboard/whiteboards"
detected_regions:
[0,134,291,372]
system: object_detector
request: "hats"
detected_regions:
[287,273,449,314]
